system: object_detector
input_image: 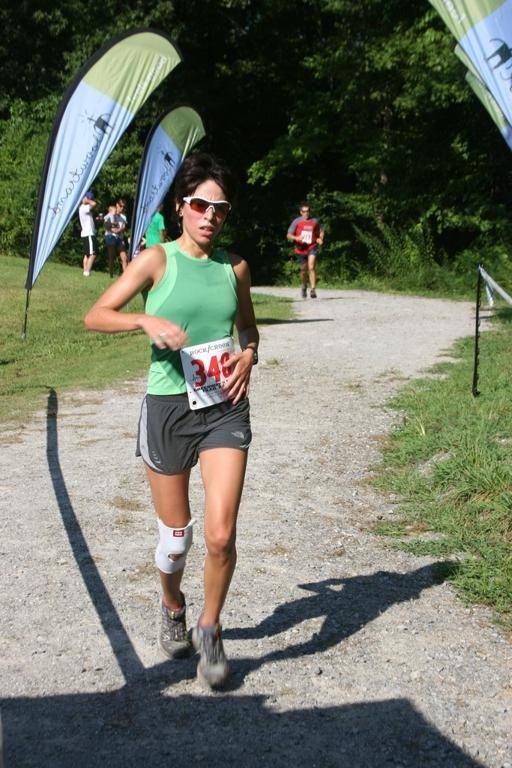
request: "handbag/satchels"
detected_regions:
[117,236,129,252]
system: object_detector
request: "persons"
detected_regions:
[103,198,132,277]
[287,204,326,298]
[78,192,99,277]
[85,145,262,690]
[126,233,147,247]
[146,204,167,248]
[106,202,117,227]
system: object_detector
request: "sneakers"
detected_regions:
[188,614,230,689]
[310,289,316,298]
[159,592,191,659]
[301,285,308,298]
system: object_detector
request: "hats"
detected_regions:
[83,191,94,201]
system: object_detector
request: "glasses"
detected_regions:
[181,196,232,220]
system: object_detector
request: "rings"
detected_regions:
[159,332,169,340]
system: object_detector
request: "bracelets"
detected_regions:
[243,345,259,365]
